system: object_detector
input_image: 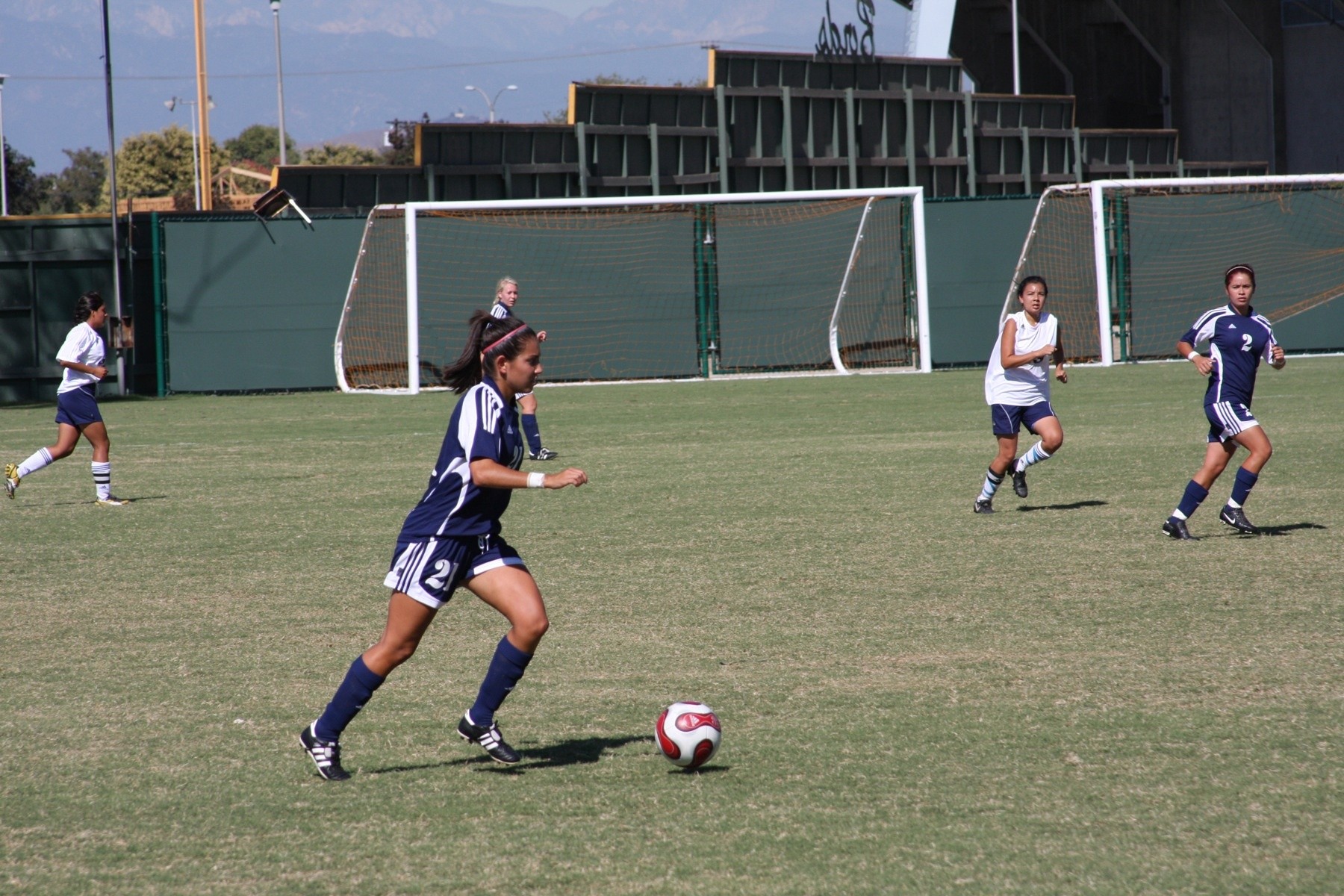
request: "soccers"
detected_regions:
[653,699,722,769]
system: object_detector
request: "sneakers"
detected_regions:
[457,709,521,766]
[4,463,22,500]
[1007,459,1028,499]
[528,446,557,461]
[94,496,129,508]
[1161,520,1200,541]
[299,720,352,782]
[1219,504,1260,535]
[973,500,993,514]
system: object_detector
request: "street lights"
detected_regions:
[464,85,519,123]
[164,94,216,211]
[269,0,286,165]
[0,73,11,216]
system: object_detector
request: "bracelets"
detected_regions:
[526,473,545,491]
[1189,349,1200,360]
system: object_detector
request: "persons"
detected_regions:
[4,292,133,505]
[1163,263,1287,541]
[973,275,1068,516]
[299,308,587,782]
[487,277,558,462]
